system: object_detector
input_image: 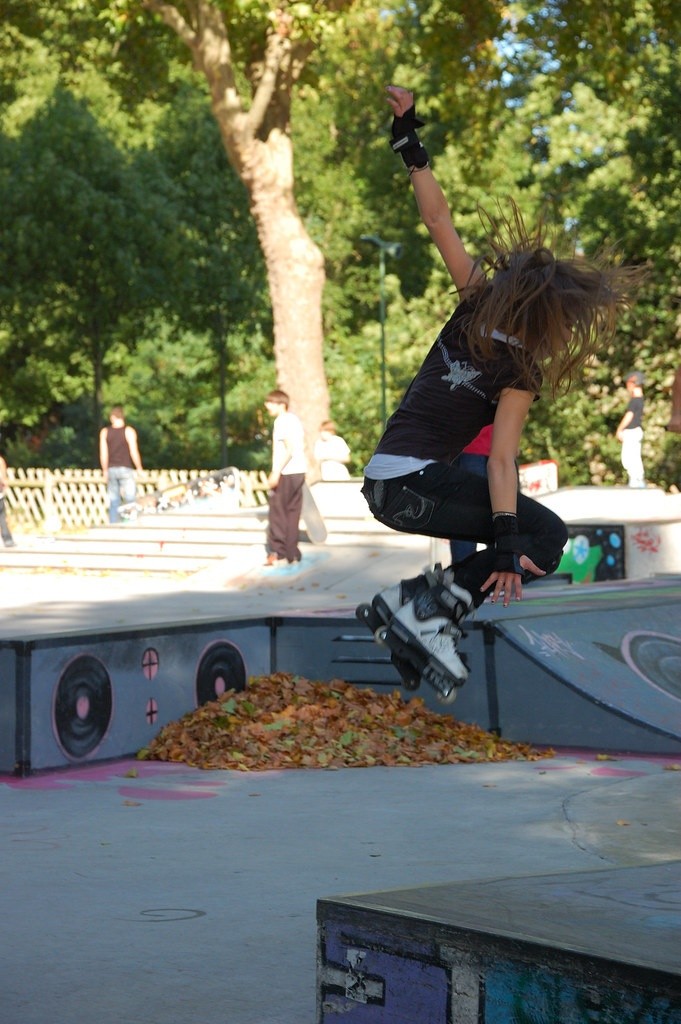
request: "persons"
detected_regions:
[616,372,645,488]
[0,456,15,547]
[356,86,652,703]
[99,406,143,523]
[265,390,306,565]
[314,421,352,480]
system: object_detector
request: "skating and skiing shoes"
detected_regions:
[358,561,456,692]
[376,568,469,704]
